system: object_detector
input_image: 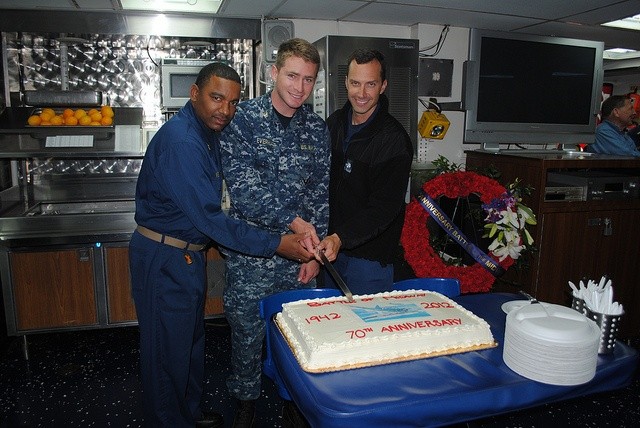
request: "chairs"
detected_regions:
[258,287,346,403]
[392,277,462,293]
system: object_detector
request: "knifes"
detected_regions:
[315,249,353,302]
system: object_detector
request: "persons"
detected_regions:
[126,61,315,427]
[320,49,415,295]
[585,95,640,157]
[215,39,330,427]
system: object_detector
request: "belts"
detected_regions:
[135,222,208,253]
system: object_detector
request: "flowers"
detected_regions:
[400,154,537,293]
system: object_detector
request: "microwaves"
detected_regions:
[161,58,229,108]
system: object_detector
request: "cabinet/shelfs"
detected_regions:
[464,148,640,339]
[0,242,237,361]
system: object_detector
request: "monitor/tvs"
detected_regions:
[463,28,604,155]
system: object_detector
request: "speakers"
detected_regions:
[260,19,294,63]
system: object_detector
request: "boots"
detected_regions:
[282,401,309,426]
[233,400,258,426]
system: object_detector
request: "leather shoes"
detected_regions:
[196,412,224,427]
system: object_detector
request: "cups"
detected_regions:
[571,298,626,355]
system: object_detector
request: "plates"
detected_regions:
[501,299,553,313]
[503,302,602,386]
